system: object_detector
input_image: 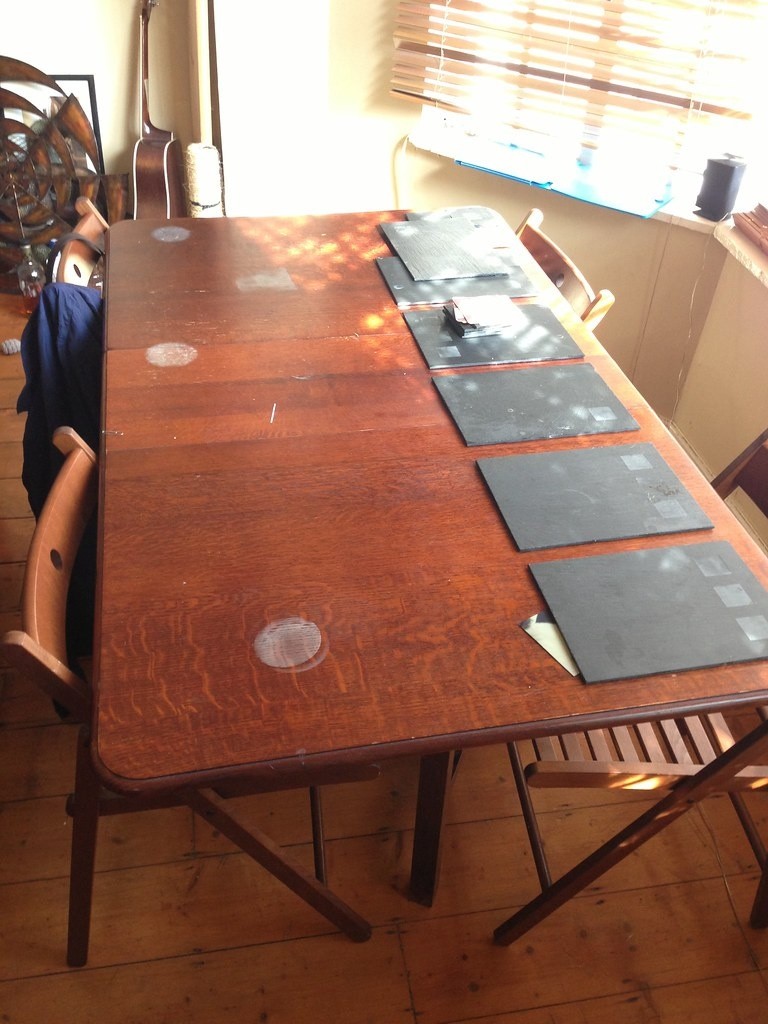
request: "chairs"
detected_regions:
[516,207,614,331]
[57,195,109,288]
[451,429,768,943]
[2,426,372,969]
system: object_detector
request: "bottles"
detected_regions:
[17,237,59,319]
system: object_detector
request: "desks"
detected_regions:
[88,204,768,906]
